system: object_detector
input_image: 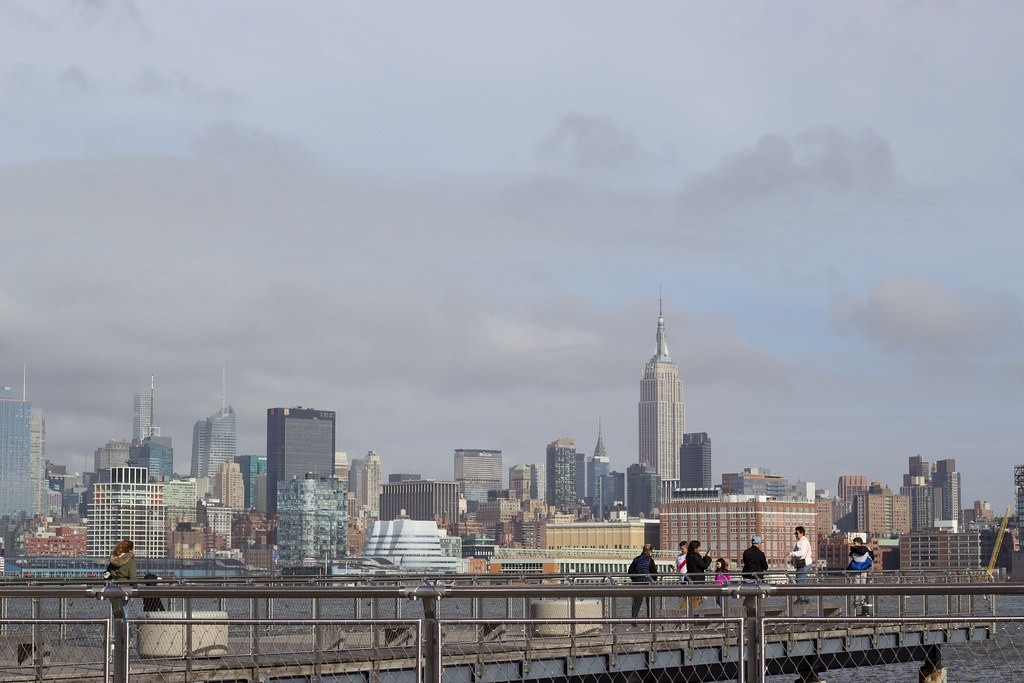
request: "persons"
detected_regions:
[675,540,692,584]
[139,572,165,612]
[103,538,137,650]
[626,543,657,628]
[788,524,814,605]
[713,557,732,607]
[846,536,875,605]
[680,538,713,611]
[740,535,769,607]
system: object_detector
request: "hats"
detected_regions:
[751,536,765,543]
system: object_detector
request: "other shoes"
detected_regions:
[853,600,867,605]
[793,599,810,605]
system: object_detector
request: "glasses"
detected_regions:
[794,532,800,535]
